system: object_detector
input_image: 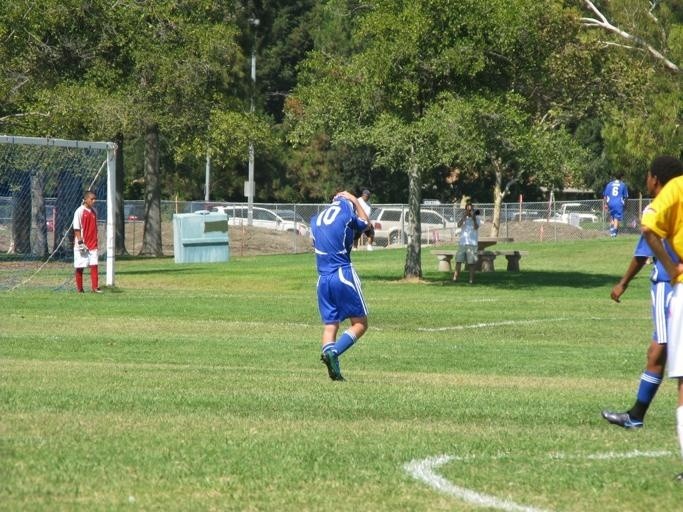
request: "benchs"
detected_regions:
[430,249,529,274]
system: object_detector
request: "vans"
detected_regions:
[367,207,462,247]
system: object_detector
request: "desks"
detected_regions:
[459,238,514,272]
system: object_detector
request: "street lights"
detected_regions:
[245,17,260,228]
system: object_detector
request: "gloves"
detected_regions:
[78,240,89,255]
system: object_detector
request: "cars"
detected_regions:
[184,200,309,241]
[512,203,599,230]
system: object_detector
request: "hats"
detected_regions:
[362,190,371,196]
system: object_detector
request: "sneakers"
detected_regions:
[320,350,341,381]
[94,289,103,293]
[601,409,643,430]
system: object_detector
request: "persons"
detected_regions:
[639,149,683,482]
[452,203,480,283]
[603,172,629,238]
[72,191,104,293]
[310,192,373,381]
[353,190,374,250]
[602,155,681,430]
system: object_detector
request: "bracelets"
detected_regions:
[78,241,83,244]
